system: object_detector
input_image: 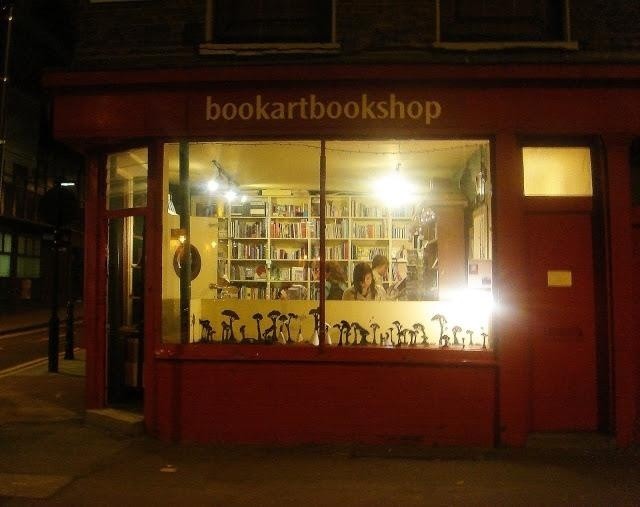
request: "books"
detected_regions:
[230,198,438,299]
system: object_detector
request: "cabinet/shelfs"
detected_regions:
[217,196,468,302]
